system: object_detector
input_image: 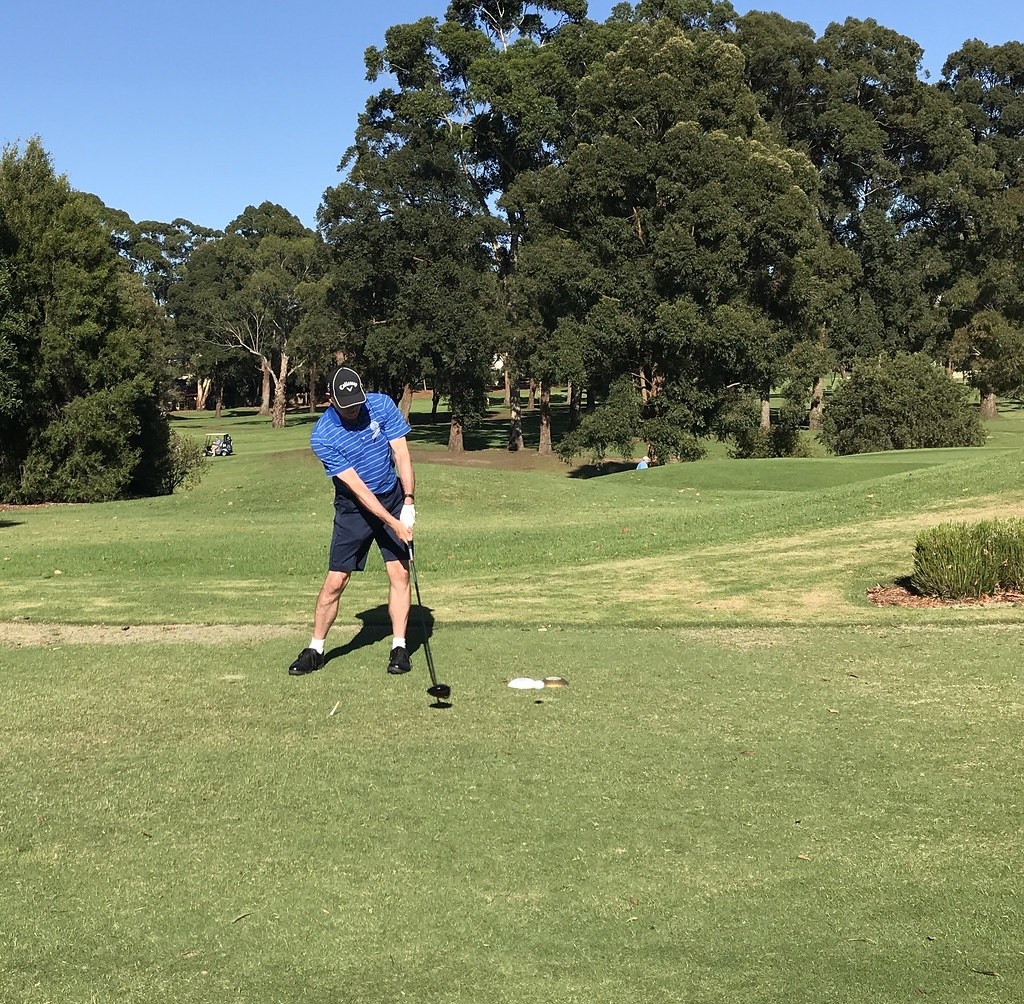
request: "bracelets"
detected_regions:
[402,494,415,501]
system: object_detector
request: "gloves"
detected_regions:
[400,504,416,529]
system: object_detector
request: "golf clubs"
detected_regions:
[408,539,451,699]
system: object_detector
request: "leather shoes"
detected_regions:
[387,646,413,674]
[288,647,325,675]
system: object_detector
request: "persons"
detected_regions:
[287,364,416,677]
[171,443,181,452]
[211,437,220,457]
[634,455,651,470]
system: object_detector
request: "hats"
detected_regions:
[330,366,368,409]
